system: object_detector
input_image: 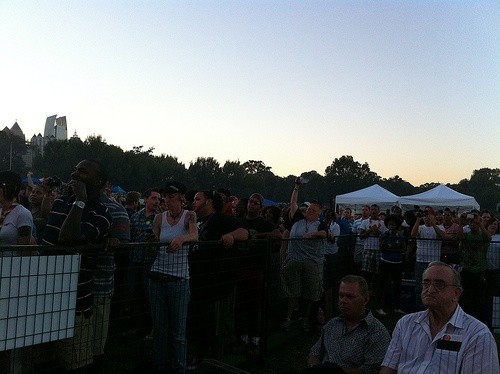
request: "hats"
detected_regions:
[249,192,265,207]
[301,201,311,208]
[165,180,187,195]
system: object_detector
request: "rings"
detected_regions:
[223,238,226,240]
[173,244,176,247]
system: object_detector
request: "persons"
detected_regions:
[379,260,500,374]
[0,160,500,374]
[305,275,391,374]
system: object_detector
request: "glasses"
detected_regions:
[442,213,451,216]
[420,279,457,290]
[248,198,260,206]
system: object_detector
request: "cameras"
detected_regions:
[417,211,429,217]
[46,175,61,187]
[466,214,474,219]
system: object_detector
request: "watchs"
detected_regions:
[73,201,85,209]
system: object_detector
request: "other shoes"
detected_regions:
[302,320,310,332]
[228,341,260,360]
[394,308,406,314]
[279,318,292,333]
[186,361,199,370]
[376,308,387,317]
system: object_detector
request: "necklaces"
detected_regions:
[424,320,446,331]
[169,208,182,221]
[347,323,356,328]
[305,220,309,233]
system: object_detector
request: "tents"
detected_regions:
[399,185,481,213]
[335,183,400,214]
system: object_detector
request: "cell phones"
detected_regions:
[146,228,153,235]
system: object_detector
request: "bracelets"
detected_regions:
[294,188,299,192]
[231,233,236,241]
